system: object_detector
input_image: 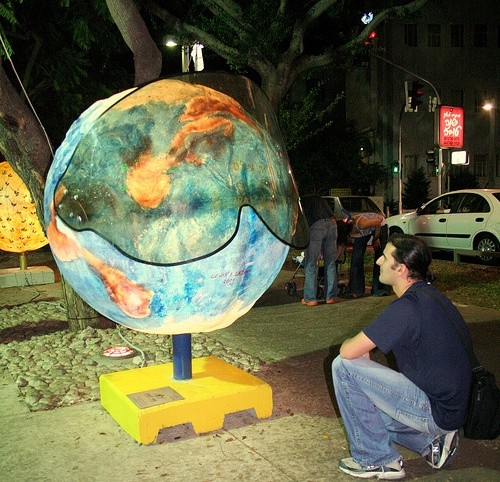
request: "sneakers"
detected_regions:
[426,430,460,468]
[340,454,405,481]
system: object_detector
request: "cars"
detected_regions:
[320,196,383,243]
[384,189,500,262]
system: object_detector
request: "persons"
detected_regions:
[435,209,451,214]
[330,233,484,480]
[293,194,340,306]
[461,205,472,213]
[335,211,392,299]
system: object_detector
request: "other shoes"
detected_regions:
[301,298,317,306]
[326,299,335,303]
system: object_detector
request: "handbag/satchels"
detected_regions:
[463,369,499,439]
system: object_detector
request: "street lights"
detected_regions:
[164,35,191,82]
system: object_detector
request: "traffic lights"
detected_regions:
[426,146,439,165]
[411,79,426,106]
[393,161,399,173]
